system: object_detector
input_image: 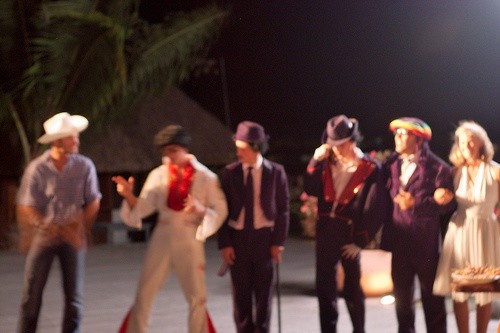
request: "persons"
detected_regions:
[16,112,103,333]
[111,125,229,333]
[432,121,500,333]
[379,117,458,333]
[303,114,387,333]
[219,121,291,333]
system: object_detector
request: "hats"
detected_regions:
[152,124,193,149]
[320,114,359,148]
[388,116,432,141]
[36,111,90,144]
[231,120,268,145]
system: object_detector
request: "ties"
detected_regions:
[243,166,254,235]
